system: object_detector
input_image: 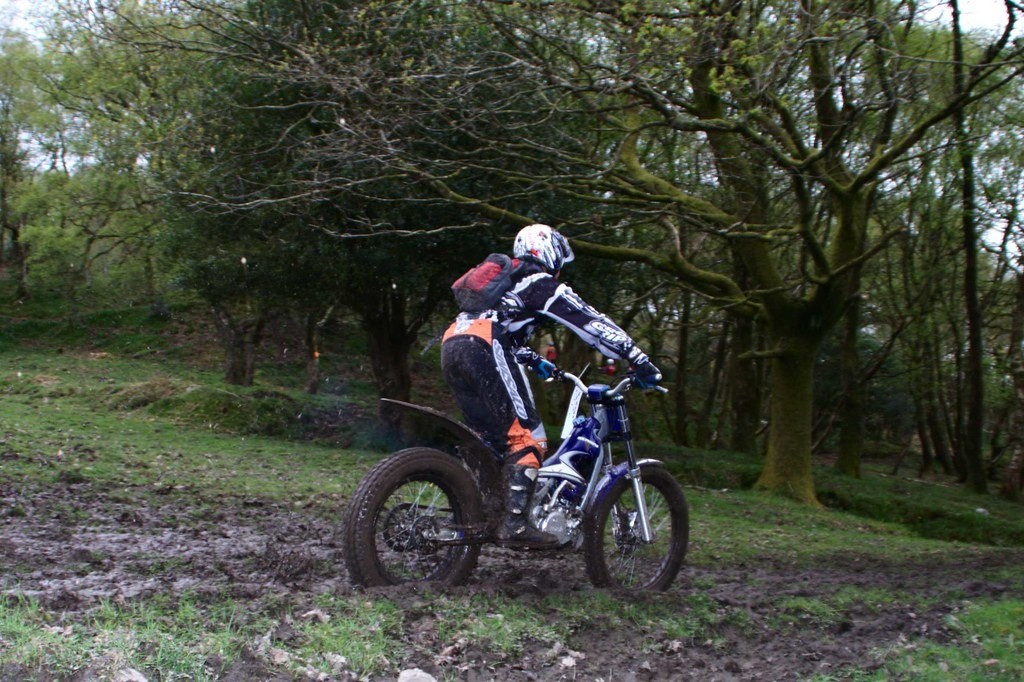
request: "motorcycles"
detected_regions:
[340,346,690,605]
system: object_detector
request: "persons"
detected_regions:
[441,224,663,549]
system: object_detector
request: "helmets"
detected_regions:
[514,223,574,272]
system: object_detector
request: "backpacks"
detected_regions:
[451,253,543,314]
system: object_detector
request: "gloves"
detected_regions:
[530,357,555,379]
[627,362,660,388]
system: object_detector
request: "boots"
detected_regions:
[496,464,561,550]
